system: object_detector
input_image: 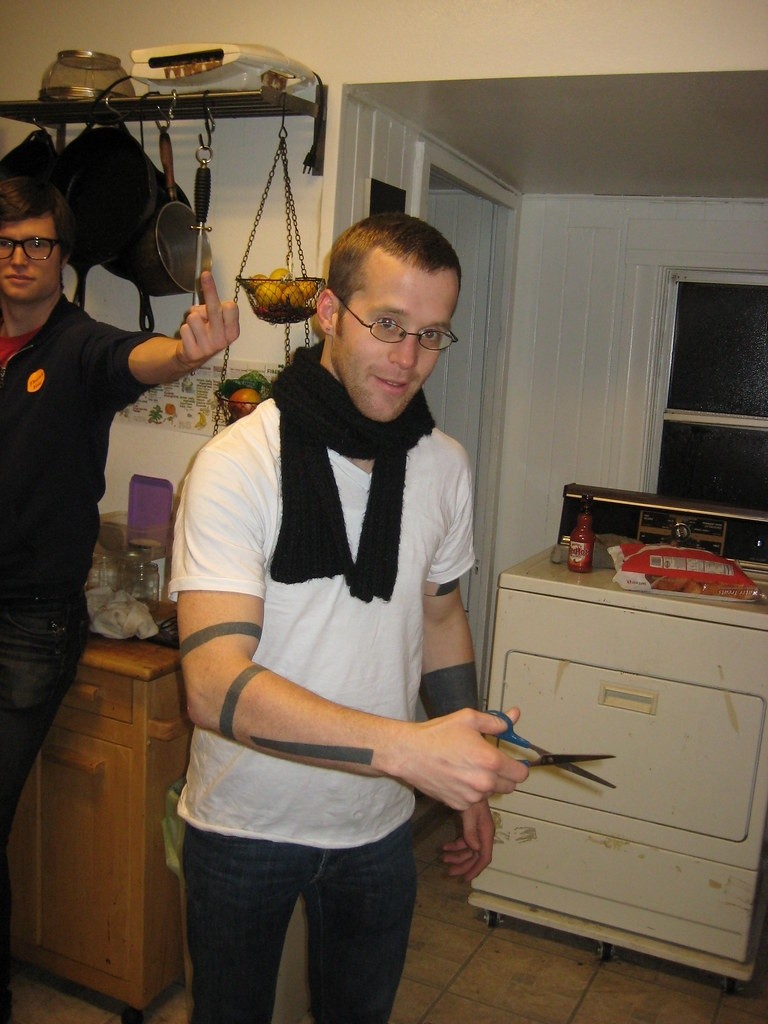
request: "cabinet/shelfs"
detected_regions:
[6,591,196,1024]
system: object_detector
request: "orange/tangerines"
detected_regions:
[249,269,316,305]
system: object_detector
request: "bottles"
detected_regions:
[568,493,594,572]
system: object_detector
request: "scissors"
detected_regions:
[484,709,618,790]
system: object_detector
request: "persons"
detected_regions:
[0,175,241,1024]
[167,213,529,1024]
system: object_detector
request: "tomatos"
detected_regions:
[229,389,261,419]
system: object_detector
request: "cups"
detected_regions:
[91,554,159,614]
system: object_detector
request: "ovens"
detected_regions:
[468,482,766,981]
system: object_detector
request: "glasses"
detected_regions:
[0,237,60,261]
[335,295,460,350]
